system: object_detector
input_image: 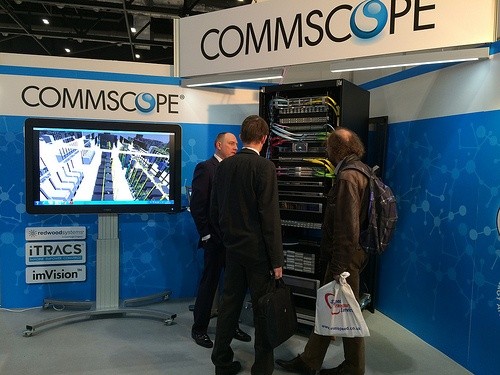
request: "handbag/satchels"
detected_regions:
[314,272,370,338]
[254,277,297,350]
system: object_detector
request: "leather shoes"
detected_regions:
[232,328,252,342]
[192,332,213,348]
[275,354,316,375]
[319,367,355,375]
[215,361,241,375]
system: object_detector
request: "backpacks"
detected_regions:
[339,163,397,257]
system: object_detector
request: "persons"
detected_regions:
[211,114,286,375]
[192,132,251,348]
[275,127,373,375]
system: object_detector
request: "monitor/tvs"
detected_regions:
[24,118,181,213]
